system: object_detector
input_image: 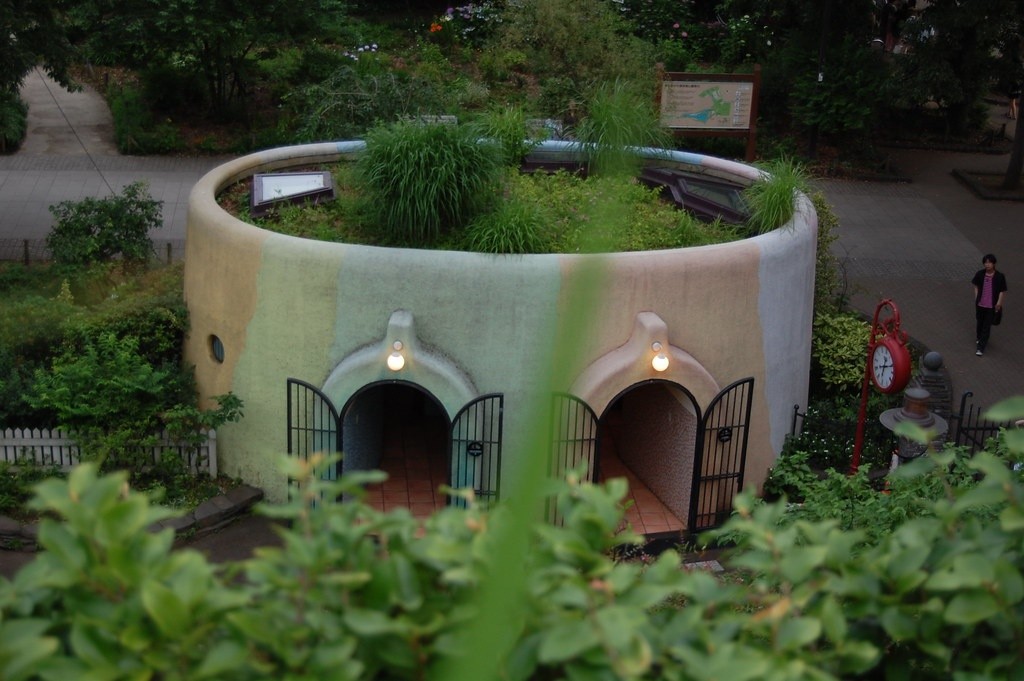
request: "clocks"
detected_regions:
[868,336,911,395]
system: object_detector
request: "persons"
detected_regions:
[1005,80,1022,120]
[970,254,1009,356]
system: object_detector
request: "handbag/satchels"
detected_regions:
[992,306,1003,326]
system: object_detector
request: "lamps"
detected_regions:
[387,340,405,371]
[652,342,669,372]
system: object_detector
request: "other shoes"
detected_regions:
[975,349,984,356]
[976,340,980,344]
